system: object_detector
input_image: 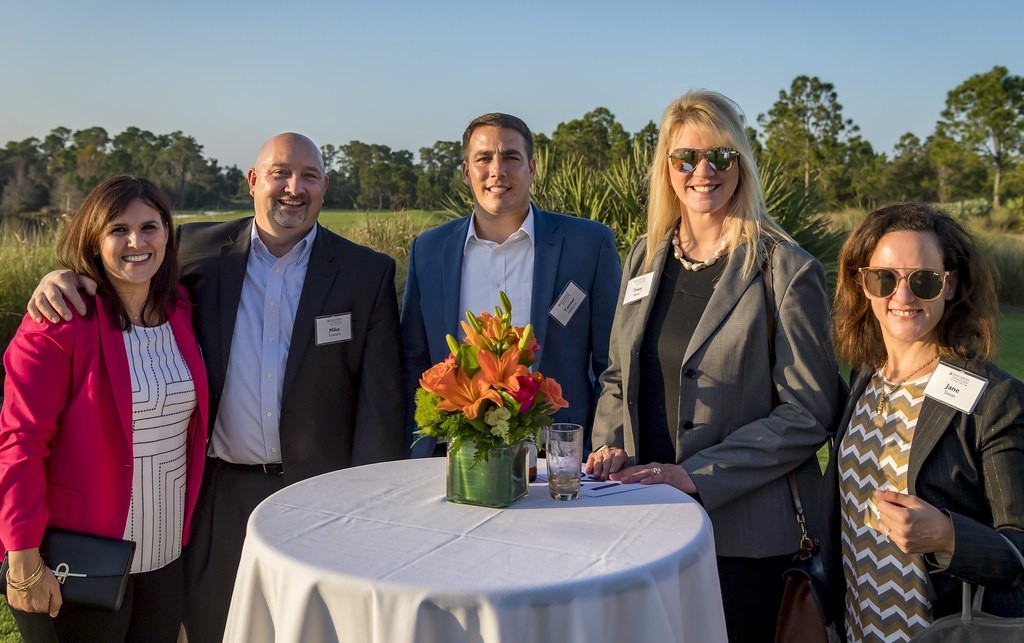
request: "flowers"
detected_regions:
[410,291,569,470]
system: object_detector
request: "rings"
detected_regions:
[654,467,661,476]
[886,528,892,542]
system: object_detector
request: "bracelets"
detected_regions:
[5,555,47,592]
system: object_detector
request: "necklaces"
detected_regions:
[876,354,940,416]
[672,228,731,272]
[122,298,147,320]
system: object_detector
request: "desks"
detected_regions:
[222,457,729,643]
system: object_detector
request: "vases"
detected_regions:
[446,434,529,508]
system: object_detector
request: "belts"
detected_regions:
[204,457,283,476]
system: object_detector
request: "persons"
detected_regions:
[26,131,400,643]
[400,112,622,459]
[1,174,210,643]
[818,201,1024,643]
[585,90,851,643]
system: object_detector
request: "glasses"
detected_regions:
[859,267,958,301]
[666,146,740,173]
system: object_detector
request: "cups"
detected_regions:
[545,423,583,501]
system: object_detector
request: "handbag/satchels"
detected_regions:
[908,532,1024,643]
[774,550,846,643]
[0,528,136,614]
